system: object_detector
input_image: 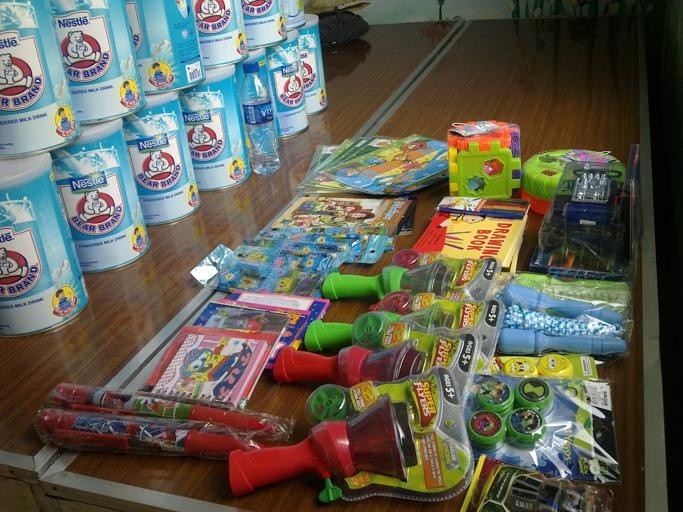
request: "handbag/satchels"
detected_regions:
[317,11,369,49]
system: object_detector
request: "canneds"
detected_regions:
[0,0,328,338]
